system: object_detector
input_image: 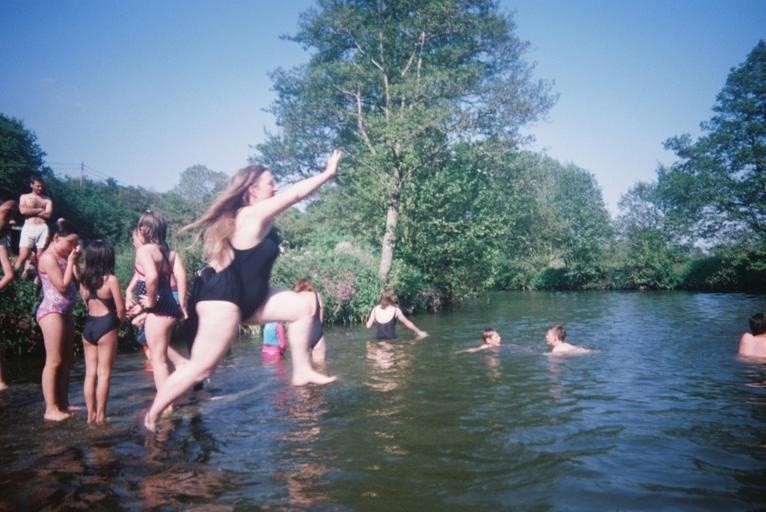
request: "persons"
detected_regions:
[544,324,597,355]
[13,176,53,286]
[262,321,287,365]
[453,327,503,353]
[737,314,766,363]
[366,287,428,338]
[294,277,325,363]
[1,194,216,424]
[136,148,343,432]
